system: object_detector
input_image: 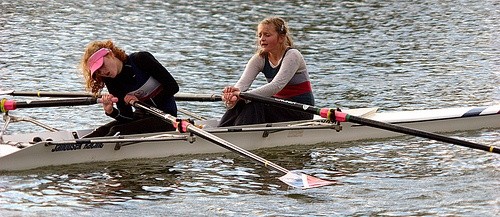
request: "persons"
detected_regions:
[82,39,179,138]
[218,17,314,127]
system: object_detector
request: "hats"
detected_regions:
[87,47,112,79]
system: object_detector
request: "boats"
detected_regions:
[0,104,500,173]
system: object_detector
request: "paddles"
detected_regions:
[230,90,500,154]
[0,90,238,101]
[0,97,118,113]
[130,100,344,191]
